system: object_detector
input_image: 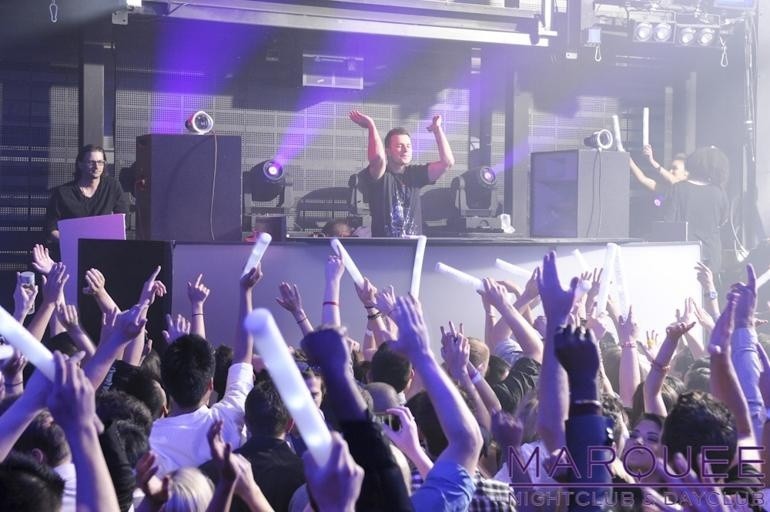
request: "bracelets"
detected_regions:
[656,164,662,173]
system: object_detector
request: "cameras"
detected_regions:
[372,412,398,431]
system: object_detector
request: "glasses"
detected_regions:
[87,160,107,166]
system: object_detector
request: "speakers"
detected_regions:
[135,133,241,242]
[531,151,630,239]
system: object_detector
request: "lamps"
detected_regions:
[632,23,718,46]
[451,167,498,210]
[242,160,290,202]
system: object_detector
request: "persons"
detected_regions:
[0,232,770,511]
[628,144,689,207]
[42,143,129,262]
[347,109,456,237]
[661,145,731,280]
